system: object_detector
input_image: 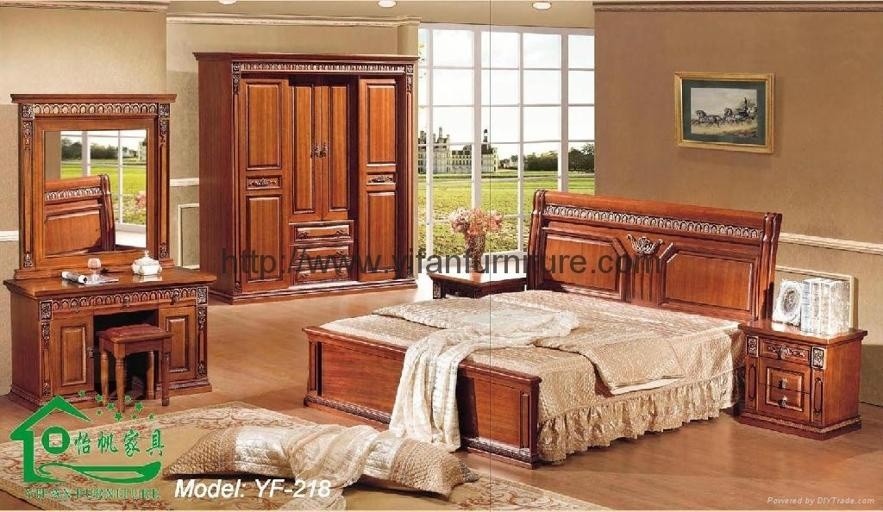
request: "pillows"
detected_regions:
[166,424,483,501]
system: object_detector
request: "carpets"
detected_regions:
[1,399,618,510]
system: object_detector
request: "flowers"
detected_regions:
[448,204,504,238]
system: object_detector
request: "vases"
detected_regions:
[463,233,487,273]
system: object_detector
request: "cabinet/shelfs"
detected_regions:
[1,267,218,417]
[191,47,421,306]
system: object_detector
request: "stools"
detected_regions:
[95,322,175,414]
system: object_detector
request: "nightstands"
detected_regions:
[735,315,869,441]
[427,267,528,297]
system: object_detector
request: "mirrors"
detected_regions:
[8,90,177,282]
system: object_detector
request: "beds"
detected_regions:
[298,188,783,472]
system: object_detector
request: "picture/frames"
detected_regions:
[671,69,775,156]
